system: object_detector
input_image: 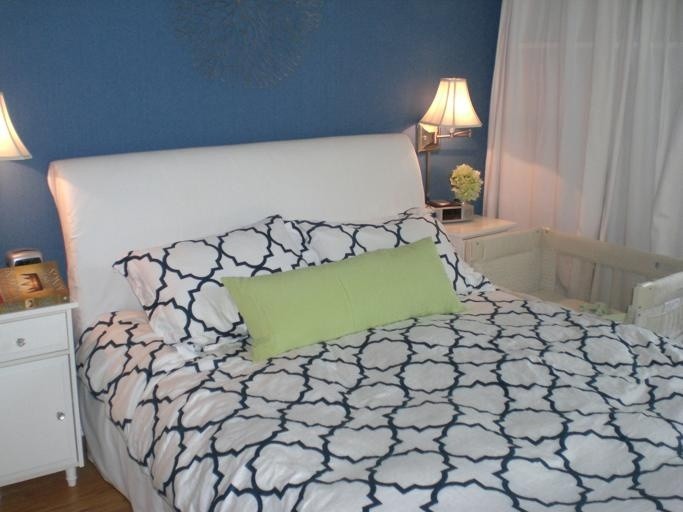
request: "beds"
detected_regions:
[468,225,682,346]
[46,132,683,511]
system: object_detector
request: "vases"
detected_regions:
[453,198,474,206]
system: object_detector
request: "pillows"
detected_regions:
[281,206,495,294]
[113,213,321,361]
[220,237,465,361]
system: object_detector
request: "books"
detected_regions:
[0,260,70,313]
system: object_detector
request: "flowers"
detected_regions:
[450,163,483,201]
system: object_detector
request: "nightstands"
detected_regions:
[0,294,85,489]
[442,213,518,262]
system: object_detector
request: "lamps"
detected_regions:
[1,91,32,161]
[416,77,484,154]
